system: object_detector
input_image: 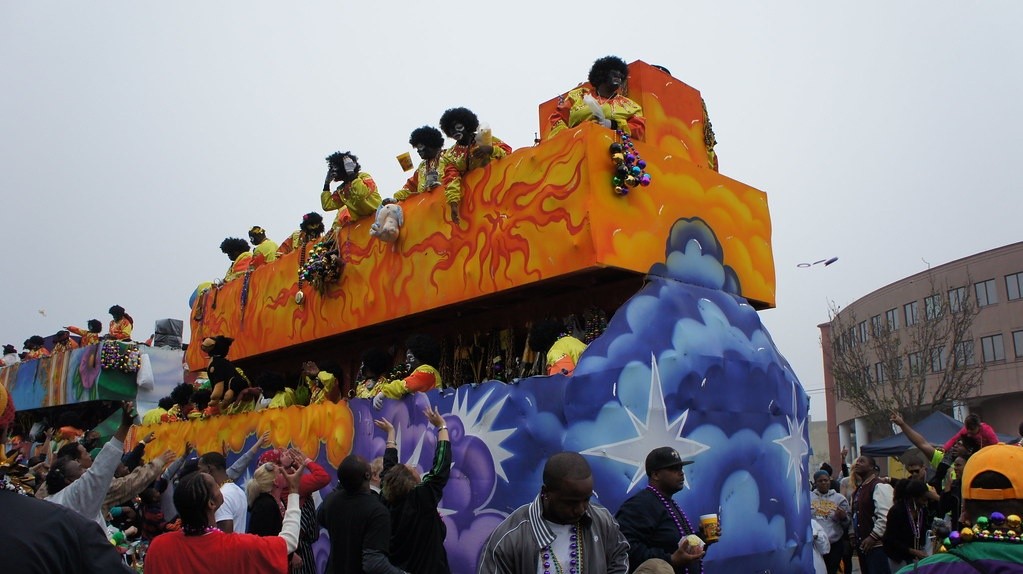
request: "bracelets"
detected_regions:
[386,441,397,445]
[438,426,448,432]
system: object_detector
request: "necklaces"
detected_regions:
[648,485,704,574]
[906,504,923,550]
[610,130,650,193]
[938,512,1023,554]
[194,226,351,327]
[182,527,223,532]
[0,480,29,496]
[541,521,584,574]
[557,309,606,341]
[54,343,141,372]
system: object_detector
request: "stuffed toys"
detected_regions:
[202,335,248,407]
[370,204,404,251]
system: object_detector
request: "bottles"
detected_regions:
[480,129,492,165]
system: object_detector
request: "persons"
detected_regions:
[615,447,723,574]
[547,56,646,139]
[218,107,512,283]
[0,305,132,366]
[143,339,443,409]
[888,407,1000,531]
[810,445,899,574]
[0,383,454,574]
[529,317,589,375]
[895,442,1023,574]
[885,478,930,574]
[478,452,630,574]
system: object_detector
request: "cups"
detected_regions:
[700,514,719,540]
[396,151,414,172]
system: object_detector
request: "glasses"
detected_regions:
[909,467,923,474]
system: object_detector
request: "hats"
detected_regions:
[53,331,70,342]
[961,442,1023,499]
[257,449,280,469]
[23,339,29,349]
[645,447,695,475]
[3,344,16,353]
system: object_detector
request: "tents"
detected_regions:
[860,411,1020,457]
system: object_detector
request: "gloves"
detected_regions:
[326,169,333,183]
[342,155,356,173]
[373,391,386,410]
